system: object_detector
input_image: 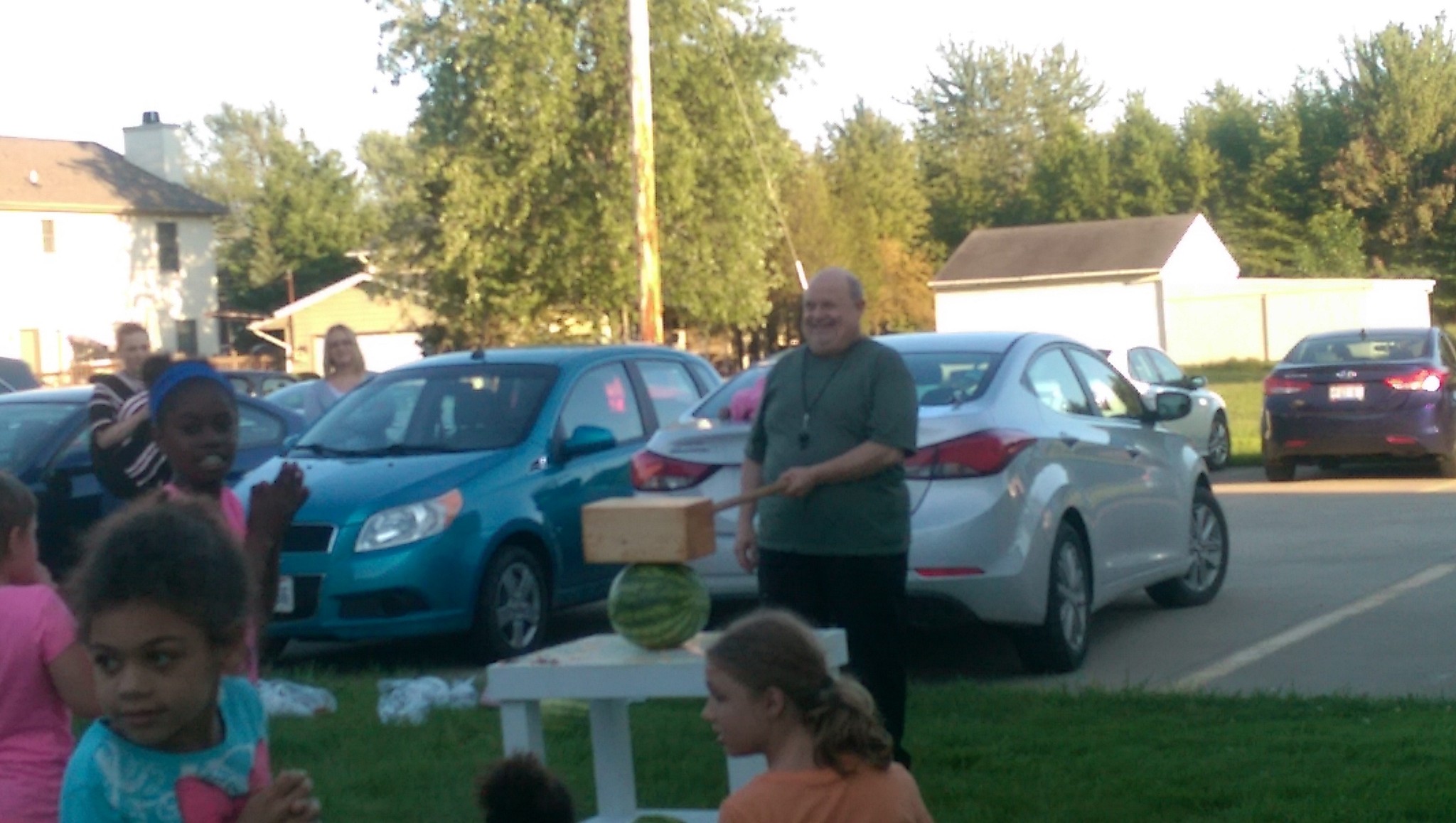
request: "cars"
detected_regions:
[1033,342,1235,471]
[617,330,1231,676]
[0,366,327,578]
[1258,325,1456,478]
[224,340,727,663]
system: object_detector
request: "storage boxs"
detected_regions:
[582,495,716,565]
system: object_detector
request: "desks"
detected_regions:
[488,626,848,823]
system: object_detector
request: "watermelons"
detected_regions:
[606,561,712,649]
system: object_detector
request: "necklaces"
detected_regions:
[797,347,855,451]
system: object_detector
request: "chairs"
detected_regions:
[447,387,516,450]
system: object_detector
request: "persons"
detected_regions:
[304,325,395,450]
[735,268,918,768]
[700,610,932,822]
[1,324,320,823]
[475,753,574,823]
